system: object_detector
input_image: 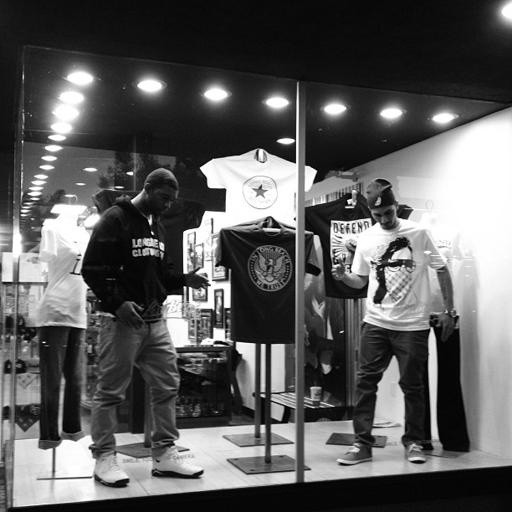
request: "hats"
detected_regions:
[366,178,395,209]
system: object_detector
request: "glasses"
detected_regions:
[387,258,417,272]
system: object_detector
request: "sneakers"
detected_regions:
[151,444,204,479]
[336,442,373,465]
[93,449,130,487]
[404,444,427,462]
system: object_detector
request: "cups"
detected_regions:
[310,387,323,407]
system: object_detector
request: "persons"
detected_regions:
[331,175,456,464]
[81,166,211,487]
[29,213,91,450]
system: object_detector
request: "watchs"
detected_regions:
[442,309,456,318]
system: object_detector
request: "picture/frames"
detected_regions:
[182,234,233,343]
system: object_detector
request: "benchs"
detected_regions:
[253,390,344,421]
